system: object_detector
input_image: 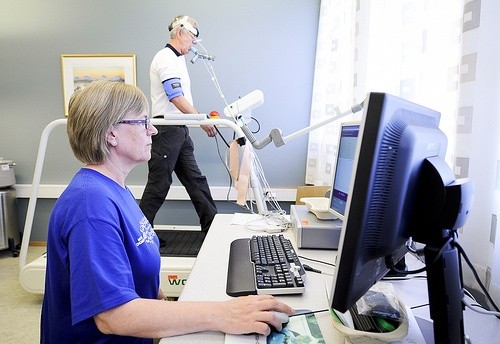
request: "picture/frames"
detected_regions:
[61,53,137,116]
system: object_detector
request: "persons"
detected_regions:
[139,17,217,248]
[40,80,295,344]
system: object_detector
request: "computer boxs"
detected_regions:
[289,205,344,250]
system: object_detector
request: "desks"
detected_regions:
[159,213,500,344]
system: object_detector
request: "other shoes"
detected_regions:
[159,237,165,248]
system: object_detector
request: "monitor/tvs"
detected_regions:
[329,94,471,344]
[328,121,361,223]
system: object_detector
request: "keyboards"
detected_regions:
[226,234,306,296]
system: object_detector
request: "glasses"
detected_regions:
[117,116,151,130]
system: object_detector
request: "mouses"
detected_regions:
[242,309,289,336]
